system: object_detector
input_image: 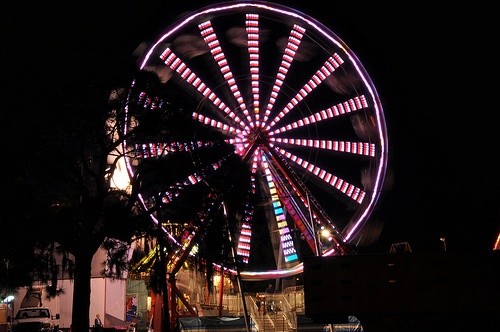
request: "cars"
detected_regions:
[6,306,61,332]
[190,303,219,318]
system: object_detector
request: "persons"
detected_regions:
[254,283,285,315]
